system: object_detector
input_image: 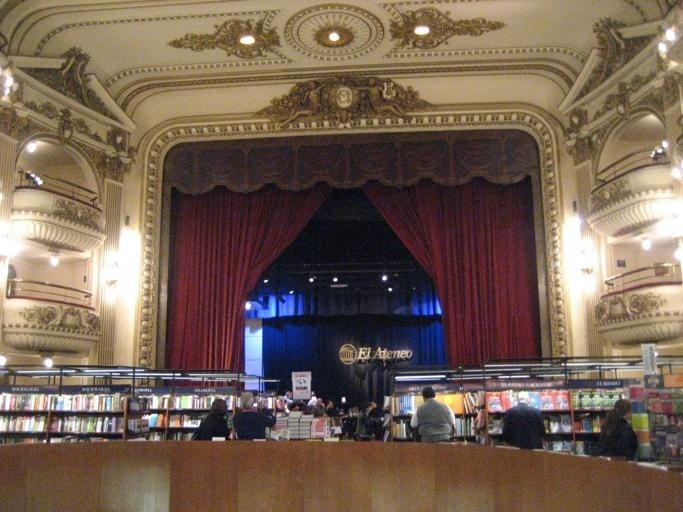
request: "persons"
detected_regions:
[277,78,333,129]
[349,77,411,123]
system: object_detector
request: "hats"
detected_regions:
[240,392,254,407]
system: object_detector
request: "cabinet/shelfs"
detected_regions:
[382,355,682,475]
[0,364,280,442]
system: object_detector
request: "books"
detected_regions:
[1,386,683,471]
